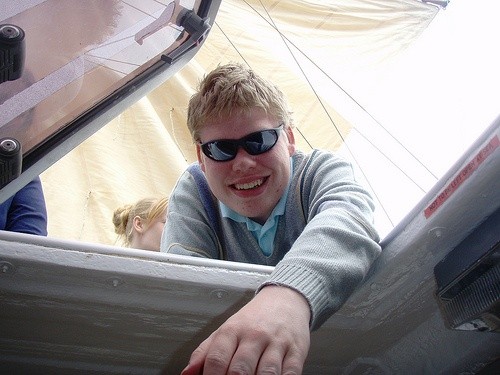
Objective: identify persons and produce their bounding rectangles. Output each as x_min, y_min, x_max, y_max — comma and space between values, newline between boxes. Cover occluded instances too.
0, 67, 49, 237
113, 194, 170, 253
160, 61, 383, 375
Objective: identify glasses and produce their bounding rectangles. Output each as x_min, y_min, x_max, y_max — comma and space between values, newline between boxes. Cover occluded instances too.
197, 123, 285, 163
